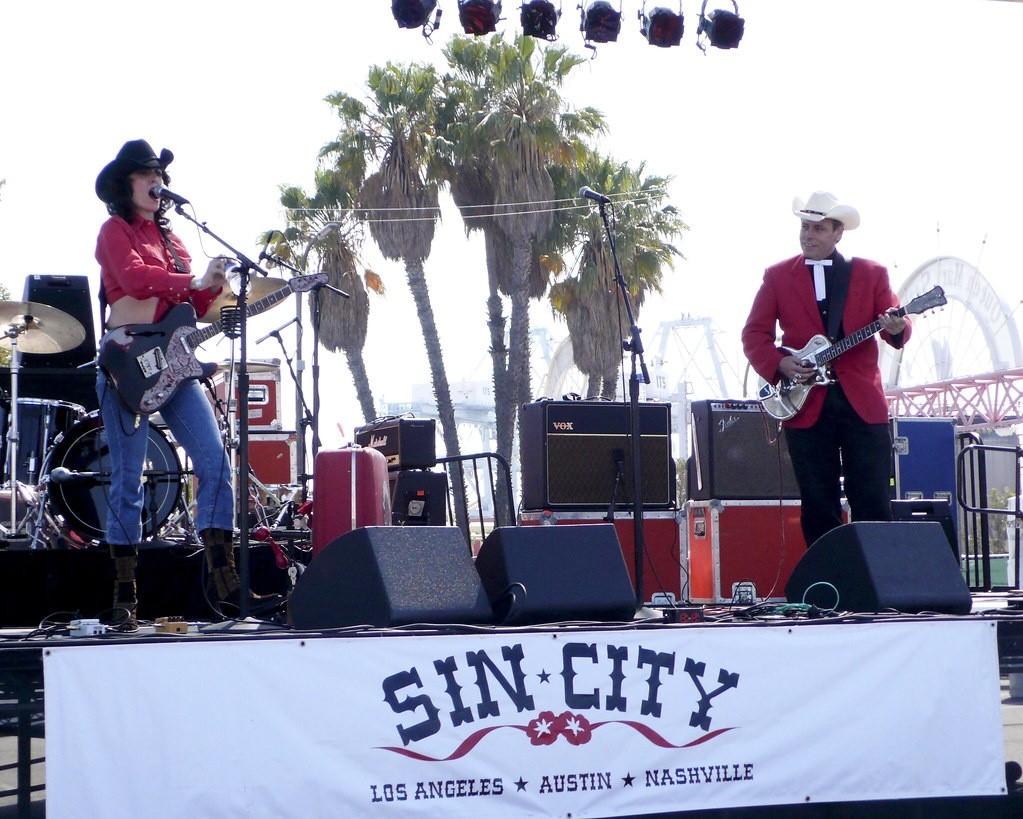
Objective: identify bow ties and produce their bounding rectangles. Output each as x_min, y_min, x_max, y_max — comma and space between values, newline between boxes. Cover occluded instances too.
804, 259, 833, 302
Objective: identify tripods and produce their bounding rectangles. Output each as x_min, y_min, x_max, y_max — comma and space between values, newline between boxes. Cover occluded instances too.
158, 375, 295, 541
0, 325, 70, 550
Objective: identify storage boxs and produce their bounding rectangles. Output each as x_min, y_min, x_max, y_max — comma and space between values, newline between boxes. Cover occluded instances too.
191, 437, 298, 521
202, 357, 284, 431
686, 497, 853, 605
518, 510, 688, 608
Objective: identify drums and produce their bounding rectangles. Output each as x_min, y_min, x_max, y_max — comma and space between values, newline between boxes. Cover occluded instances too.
42, 408, 184, 543
3, 397, 87, 503
235, 482, 310, 515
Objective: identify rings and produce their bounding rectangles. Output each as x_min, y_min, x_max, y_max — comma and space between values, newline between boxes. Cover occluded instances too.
795, 373, 801, 378
793, 378, 798, 384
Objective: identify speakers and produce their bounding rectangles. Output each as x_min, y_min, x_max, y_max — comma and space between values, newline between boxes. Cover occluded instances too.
888, 499, 960, 571
691, 399, 804, 501
387, 470, 448, 527
522, 401, 672, 509
17, 275, 97, 368
473, 523, 636, 627
286, 525, 494, 631
785, 520, 973, 616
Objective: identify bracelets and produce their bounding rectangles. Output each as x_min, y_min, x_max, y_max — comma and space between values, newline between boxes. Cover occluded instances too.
196, 279, 202, 289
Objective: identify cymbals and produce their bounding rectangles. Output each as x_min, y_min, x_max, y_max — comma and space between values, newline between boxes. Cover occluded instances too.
0, 299, 88, 356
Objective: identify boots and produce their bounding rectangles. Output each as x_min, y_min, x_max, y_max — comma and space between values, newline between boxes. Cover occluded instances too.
110, 545, 140, 632
201, 530, 283, 623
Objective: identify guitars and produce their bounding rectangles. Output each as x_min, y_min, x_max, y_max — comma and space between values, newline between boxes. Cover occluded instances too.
756, 284, 949, 422
99, 271, 332, 416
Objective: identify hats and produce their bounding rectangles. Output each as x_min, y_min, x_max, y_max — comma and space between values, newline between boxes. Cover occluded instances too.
95, 139, 174, 204
792, 191, 860, 230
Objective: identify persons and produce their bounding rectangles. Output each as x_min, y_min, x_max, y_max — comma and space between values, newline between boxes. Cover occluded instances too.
95, 139, 280, 630
742, 192, 911, 550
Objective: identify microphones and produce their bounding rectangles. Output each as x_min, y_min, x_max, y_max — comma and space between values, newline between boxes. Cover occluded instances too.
154, 184, 189, 205
51, 467, 72, 483
612, 446, 626, 481
257, 232, 273, 265
256, 317, 297, 344
579, 186, 610, 202
